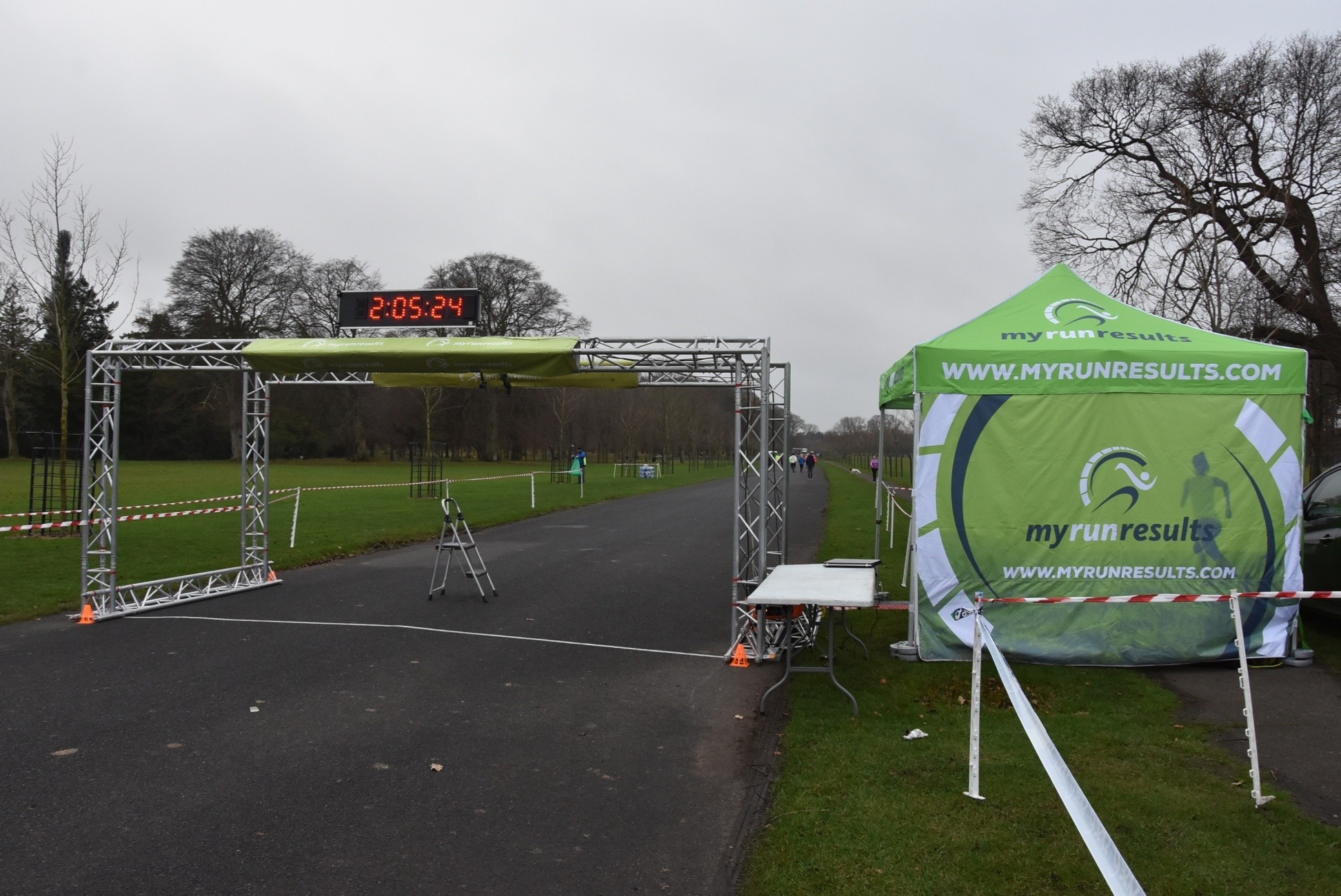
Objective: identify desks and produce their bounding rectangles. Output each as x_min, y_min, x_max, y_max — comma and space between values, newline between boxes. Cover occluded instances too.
746, 562, 875, 714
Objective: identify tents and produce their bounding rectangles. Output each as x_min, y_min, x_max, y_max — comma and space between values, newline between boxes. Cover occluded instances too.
875, 262, 1314, 670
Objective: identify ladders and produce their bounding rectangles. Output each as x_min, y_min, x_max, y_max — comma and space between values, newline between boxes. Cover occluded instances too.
429, 498, 498, 603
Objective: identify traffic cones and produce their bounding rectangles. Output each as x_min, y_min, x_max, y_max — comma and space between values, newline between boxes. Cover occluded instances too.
76, 604, 97, 625
728, 640, 752, 667
268, 570, 277, 581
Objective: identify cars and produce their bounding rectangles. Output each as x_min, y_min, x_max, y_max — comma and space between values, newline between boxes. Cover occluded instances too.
1300, 461, 1340, 620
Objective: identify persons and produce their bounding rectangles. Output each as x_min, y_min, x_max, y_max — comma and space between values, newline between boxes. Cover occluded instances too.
849, 468, 861, 475
870, 455, 879, 482
789, 451, 817, 474
571, 447, 586, 484
1179, 451, 1238, 579
806, 452, 815, 480
769, 453, 783, 468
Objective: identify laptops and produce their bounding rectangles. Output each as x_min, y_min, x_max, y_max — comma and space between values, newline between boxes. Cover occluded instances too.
823, 558, 883, 568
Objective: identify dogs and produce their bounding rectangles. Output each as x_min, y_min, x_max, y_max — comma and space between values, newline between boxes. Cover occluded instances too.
849, 468, 862, 475
